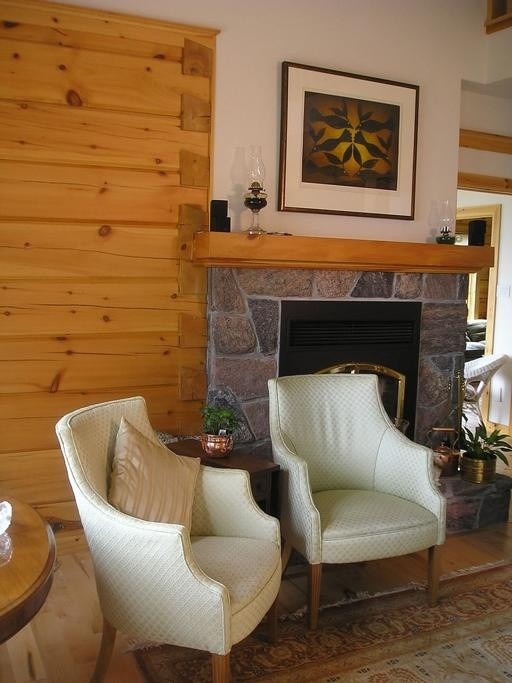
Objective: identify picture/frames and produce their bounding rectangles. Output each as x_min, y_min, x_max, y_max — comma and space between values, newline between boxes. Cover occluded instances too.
278, 61, 420, 220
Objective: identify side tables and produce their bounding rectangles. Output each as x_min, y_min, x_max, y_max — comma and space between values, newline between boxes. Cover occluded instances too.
162, 438, 281, 516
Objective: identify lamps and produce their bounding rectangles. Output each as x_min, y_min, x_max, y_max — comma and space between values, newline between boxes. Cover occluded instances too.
437, 200, 456, 245
241, 146, 269, 234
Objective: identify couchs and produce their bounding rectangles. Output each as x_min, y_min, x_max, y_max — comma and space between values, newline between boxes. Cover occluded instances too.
466, 319, 487, 342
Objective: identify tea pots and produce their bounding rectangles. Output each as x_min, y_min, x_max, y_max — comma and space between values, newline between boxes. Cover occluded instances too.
425, 428, 461, 475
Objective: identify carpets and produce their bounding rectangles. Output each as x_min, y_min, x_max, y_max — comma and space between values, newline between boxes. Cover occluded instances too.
125, 556, 512, 683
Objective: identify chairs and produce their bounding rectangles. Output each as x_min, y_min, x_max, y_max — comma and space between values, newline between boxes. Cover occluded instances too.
55, 396, 282, 683
267, 374, 448, 630
464, 353, 509, 425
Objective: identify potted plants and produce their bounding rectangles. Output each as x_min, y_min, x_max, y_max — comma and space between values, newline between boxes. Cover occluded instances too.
200, 405, 242, 459
458, 421, 512, 484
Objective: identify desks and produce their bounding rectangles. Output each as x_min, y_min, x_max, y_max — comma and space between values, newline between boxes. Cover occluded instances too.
0, 494, 57, 645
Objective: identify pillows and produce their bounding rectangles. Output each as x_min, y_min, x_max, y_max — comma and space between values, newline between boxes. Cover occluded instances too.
108, 416, 201, 532
